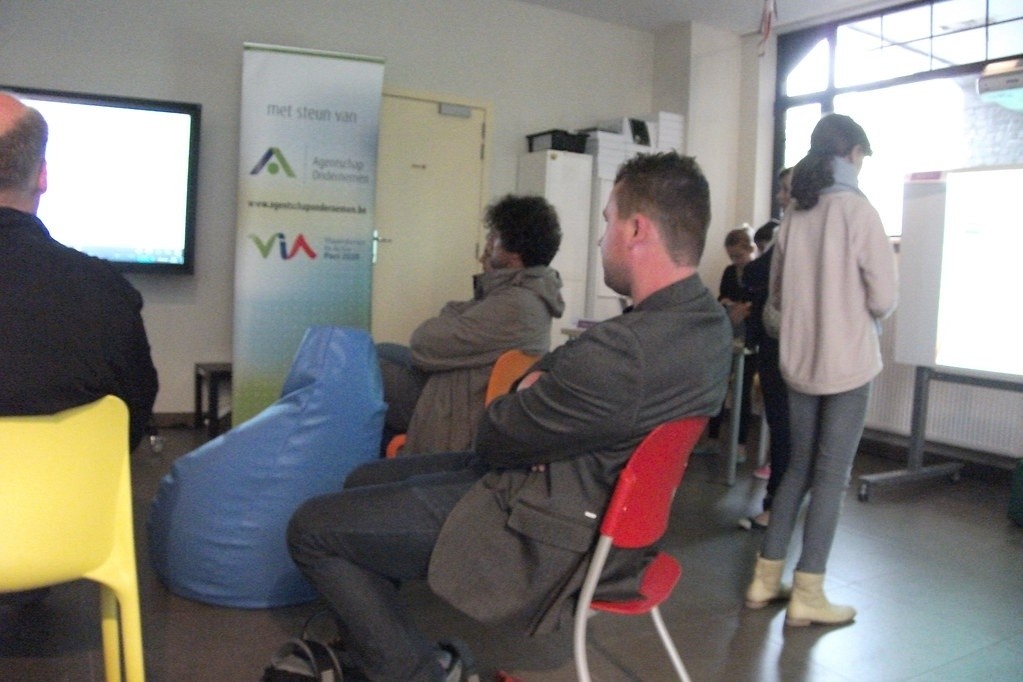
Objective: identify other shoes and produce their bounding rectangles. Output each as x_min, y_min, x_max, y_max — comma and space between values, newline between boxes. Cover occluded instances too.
739, 515, 767, 532
736, 451, 746, 464
694, 444, 720, 454
752, 464, 771, 480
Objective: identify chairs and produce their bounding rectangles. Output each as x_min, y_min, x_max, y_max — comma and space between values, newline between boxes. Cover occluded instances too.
487, 414, 713, 682
0, 395, 145, 682
386, 347, 551, 462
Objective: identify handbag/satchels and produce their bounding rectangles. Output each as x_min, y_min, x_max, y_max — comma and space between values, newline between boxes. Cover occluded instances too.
261, 611, 479, 682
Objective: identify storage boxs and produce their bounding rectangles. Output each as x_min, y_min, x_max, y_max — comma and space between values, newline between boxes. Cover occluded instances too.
516, 110, 682, 353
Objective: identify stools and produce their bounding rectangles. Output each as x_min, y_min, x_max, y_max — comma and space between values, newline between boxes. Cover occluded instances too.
193, 364, 235, 443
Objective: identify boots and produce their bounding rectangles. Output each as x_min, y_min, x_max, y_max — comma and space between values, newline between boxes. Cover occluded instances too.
786, 572, 856, 628
745, 552, 792, 608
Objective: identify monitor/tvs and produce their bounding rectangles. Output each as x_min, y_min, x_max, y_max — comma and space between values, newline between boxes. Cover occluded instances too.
0, 86, 203, 276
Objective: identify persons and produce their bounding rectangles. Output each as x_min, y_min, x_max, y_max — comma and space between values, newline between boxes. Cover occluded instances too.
287, 152, 734, 682
379, 196, 565, 456
745, 114, 900, 627
717, 165, 795, 531
0, 91, 159, 658
